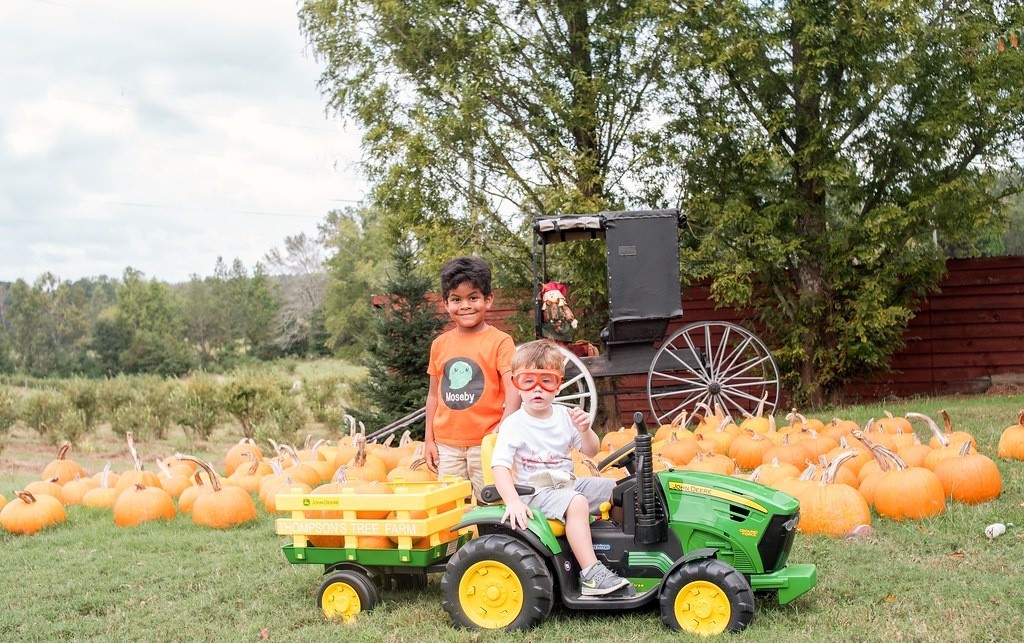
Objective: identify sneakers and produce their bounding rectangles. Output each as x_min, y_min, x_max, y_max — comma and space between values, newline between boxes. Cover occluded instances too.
580, 560, 632, 594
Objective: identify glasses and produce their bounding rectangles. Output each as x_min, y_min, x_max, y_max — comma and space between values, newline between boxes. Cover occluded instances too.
511, 370, 563, 392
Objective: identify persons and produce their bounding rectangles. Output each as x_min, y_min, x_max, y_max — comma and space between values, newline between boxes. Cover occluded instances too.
490, 339, 631, 596
423, 258, 515, 509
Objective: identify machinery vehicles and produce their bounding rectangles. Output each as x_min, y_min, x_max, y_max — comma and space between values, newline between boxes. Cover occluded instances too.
270, 409, 822, 642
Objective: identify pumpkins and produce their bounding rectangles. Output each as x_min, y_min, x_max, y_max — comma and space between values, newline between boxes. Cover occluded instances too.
0, 408, 1024, 551
